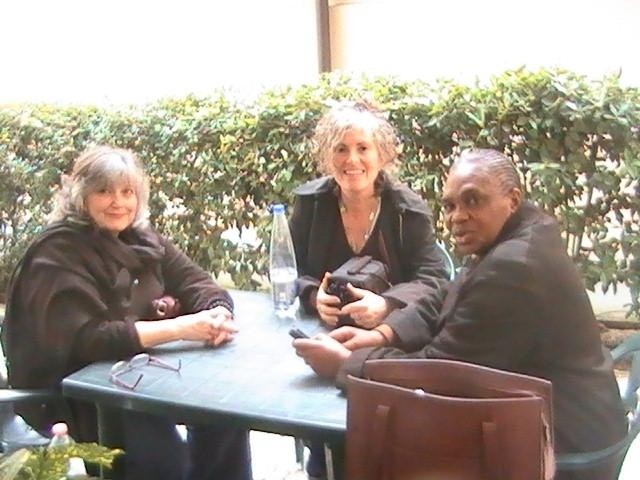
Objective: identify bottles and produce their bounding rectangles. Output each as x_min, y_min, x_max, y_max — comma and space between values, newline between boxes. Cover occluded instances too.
48, 422, 87, 479
269, 205, 301, 315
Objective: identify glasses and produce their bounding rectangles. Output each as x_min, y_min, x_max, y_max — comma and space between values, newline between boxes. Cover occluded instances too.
107, 352, 182, 390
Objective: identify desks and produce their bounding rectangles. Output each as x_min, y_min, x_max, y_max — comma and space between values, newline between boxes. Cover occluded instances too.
58, 286, 350, 479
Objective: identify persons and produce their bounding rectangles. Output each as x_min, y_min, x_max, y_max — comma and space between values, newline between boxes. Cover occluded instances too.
286, 95, 451, 478
291, 146, 630, 454
0, 145, 250, 480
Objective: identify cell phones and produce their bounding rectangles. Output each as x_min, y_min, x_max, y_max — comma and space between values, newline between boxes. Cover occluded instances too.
289, 324, 311, 337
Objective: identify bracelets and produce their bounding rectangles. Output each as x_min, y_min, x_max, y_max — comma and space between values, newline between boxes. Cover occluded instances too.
374, 327, 389, 345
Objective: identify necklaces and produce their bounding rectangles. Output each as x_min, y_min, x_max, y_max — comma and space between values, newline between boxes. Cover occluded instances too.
339, 195, 378, 252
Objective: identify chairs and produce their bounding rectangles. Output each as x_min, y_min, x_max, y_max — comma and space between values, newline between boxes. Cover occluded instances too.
553, 332, 640, 480
0, 313, 124, 478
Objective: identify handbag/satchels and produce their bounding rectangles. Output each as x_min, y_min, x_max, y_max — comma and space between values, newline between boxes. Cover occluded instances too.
343, 358, 556, 480
326, 256, 389, 326
